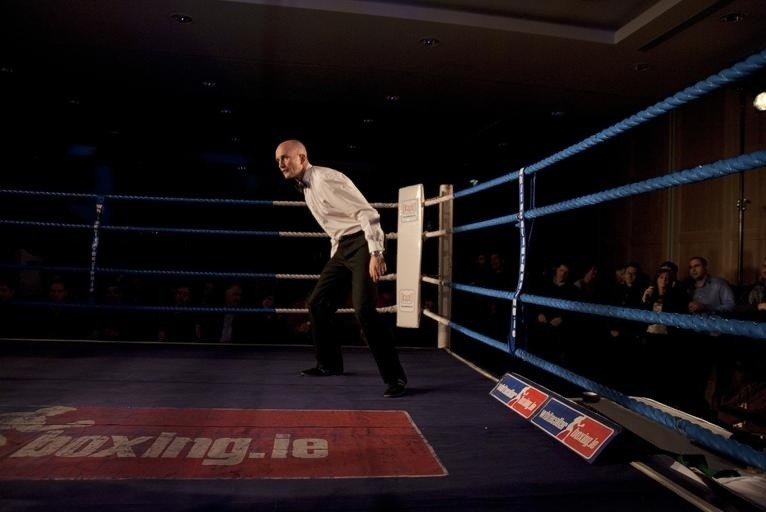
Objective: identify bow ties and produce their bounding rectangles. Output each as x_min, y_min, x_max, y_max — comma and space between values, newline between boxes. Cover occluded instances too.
295, 182, 310, 192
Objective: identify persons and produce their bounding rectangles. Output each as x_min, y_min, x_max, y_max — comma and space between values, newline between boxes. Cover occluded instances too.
274, 139, 409, 398
0, 251, 399, 351
422, 252, 765, 450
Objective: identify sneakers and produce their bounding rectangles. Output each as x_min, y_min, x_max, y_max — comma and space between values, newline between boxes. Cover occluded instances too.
302, 365, 343, 376
384, 377, 407, 396
